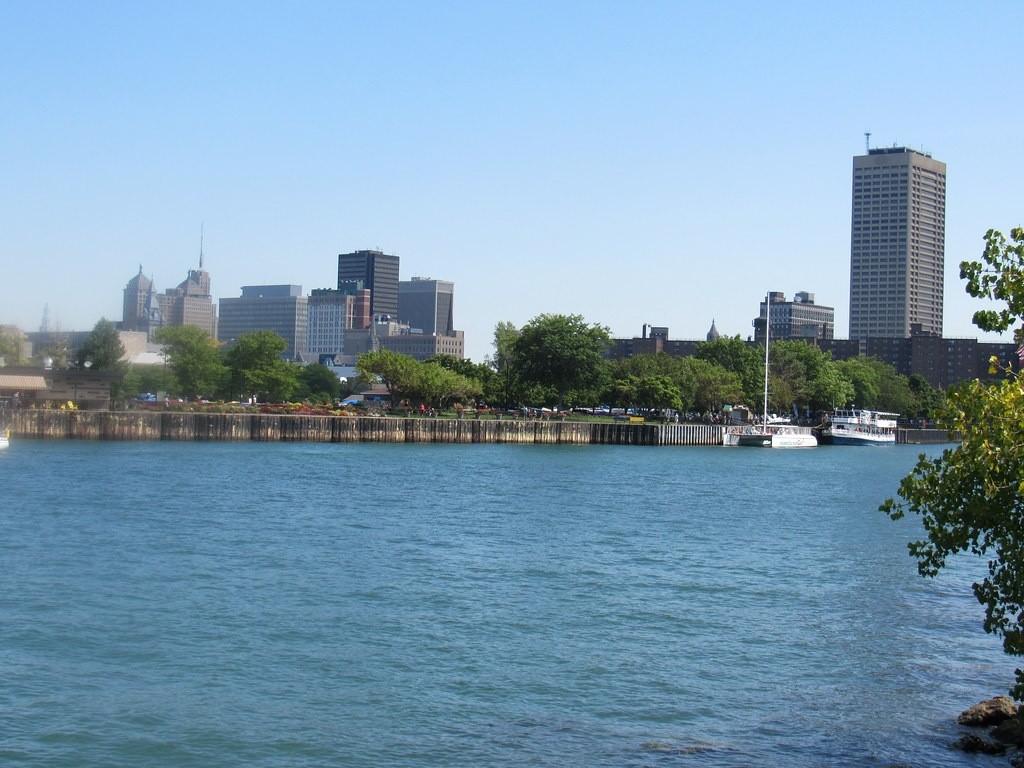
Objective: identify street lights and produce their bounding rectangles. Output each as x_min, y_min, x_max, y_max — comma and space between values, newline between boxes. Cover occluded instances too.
228, 336, 244, 404
497, 352, 508, 410
73, 360, 96, 408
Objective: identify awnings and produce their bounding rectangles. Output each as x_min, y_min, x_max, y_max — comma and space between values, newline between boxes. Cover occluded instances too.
0, 375, 48, 391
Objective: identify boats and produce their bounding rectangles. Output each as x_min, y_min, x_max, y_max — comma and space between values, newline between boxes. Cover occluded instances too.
0, 427, 9, 451
820, 404, 900, 446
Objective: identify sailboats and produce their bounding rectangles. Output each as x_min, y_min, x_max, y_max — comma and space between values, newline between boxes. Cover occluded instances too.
740, 291, 819, 448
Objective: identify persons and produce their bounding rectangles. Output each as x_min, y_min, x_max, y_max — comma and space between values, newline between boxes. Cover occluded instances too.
13, 393, 26, 411
418, 402, 432, 416
674, 412, 731, 425
253, 395, 257, 407
163, 394, 170, 411
